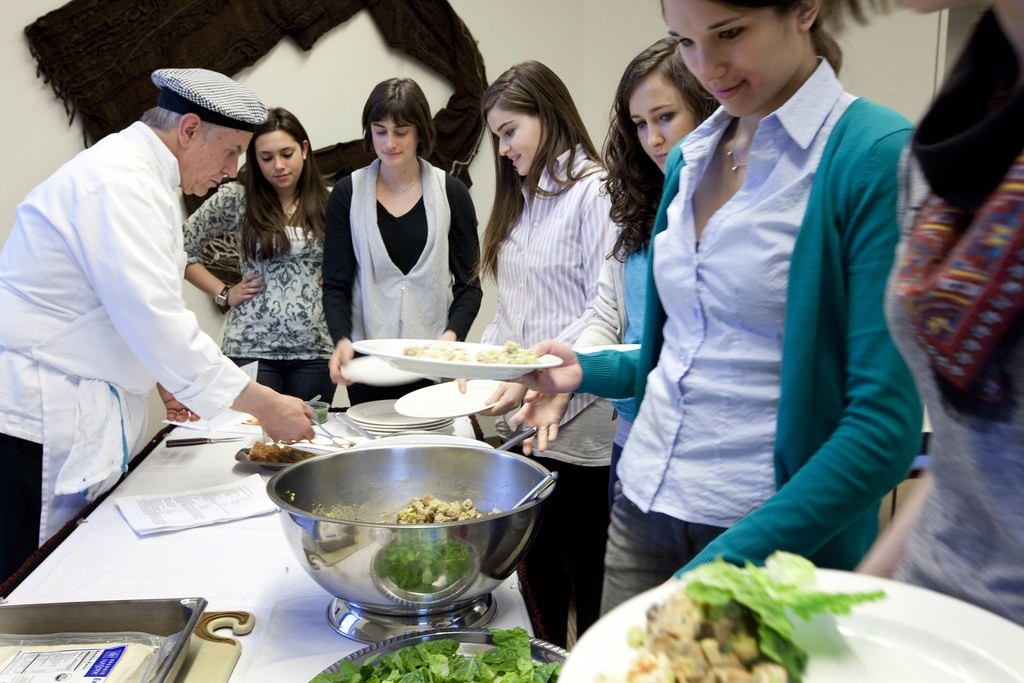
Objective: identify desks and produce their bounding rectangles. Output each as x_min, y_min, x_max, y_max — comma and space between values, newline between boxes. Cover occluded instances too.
0, 406, 540, 683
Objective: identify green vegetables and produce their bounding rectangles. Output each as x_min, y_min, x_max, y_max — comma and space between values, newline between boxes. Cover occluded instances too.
310, 628, 562, 683
376, 541, 472, 593
688, 554, 886, 682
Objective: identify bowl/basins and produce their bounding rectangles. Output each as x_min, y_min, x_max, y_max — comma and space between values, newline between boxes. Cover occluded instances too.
297, 401, 331, 424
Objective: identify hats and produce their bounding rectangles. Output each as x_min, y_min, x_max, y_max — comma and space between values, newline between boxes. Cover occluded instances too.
151, 68, 270, 134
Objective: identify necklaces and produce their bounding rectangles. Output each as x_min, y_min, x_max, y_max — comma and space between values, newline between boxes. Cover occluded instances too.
727, 132, 748, 174
378, 174, 420, 194
280, 200, 294, 216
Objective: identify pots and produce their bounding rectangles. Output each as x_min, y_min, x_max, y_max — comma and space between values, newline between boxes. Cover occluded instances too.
267, 443, 560, 645
305, 627, 574, 683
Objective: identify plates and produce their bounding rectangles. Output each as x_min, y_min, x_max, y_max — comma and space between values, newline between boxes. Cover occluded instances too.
351, 435, 495, 452
556, 562, 1024, 683
395, 380, 510, 418
346, 399, 456, 441
235, 443, 348, 466
350, 339, 566, 380
341, 357, 423, 386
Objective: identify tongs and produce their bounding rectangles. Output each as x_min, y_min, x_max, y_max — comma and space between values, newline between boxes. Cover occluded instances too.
304, 420, 356, 451
495, 422, 538, 451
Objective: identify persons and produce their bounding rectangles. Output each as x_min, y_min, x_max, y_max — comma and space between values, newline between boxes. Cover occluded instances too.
1, 69, 316, 603
840, 0, 1023, 627
458, 0, 916, 623
182, 108, 329, 402
322, 77, 483, 404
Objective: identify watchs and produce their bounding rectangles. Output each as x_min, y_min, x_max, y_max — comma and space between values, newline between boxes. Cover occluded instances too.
215, 285, 230, 315
